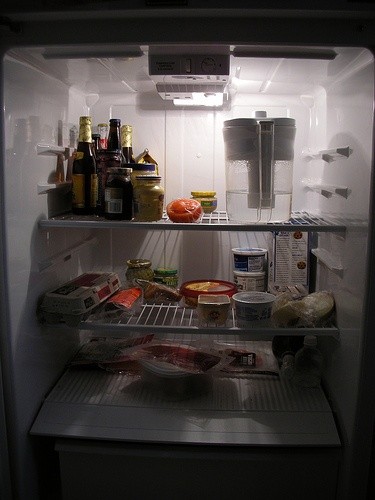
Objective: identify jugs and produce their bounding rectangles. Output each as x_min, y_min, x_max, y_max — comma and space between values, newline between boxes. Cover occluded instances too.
222, 109, 297, 224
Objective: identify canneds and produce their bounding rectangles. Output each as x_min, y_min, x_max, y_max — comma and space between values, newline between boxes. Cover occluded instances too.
103, 168, 135, 221
152, 268, 178, 288
190, 191, 217, 213
131, 163, 163, 222
125, 258, 152, 289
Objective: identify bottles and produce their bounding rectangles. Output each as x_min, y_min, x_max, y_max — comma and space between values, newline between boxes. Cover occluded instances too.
70, 115, 99, 215
91, 123, 134, 163
99, 118, 126, 219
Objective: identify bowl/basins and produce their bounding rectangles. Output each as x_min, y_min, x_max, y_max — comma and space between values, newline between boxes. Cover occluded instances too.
136, 357, 205, 395
181, 280, 237, 309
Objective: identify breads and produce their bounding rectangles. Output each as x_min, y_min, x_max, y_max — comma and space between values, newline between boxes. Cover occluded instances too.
270, 290, 335, 328
143, 282, 182, 303
